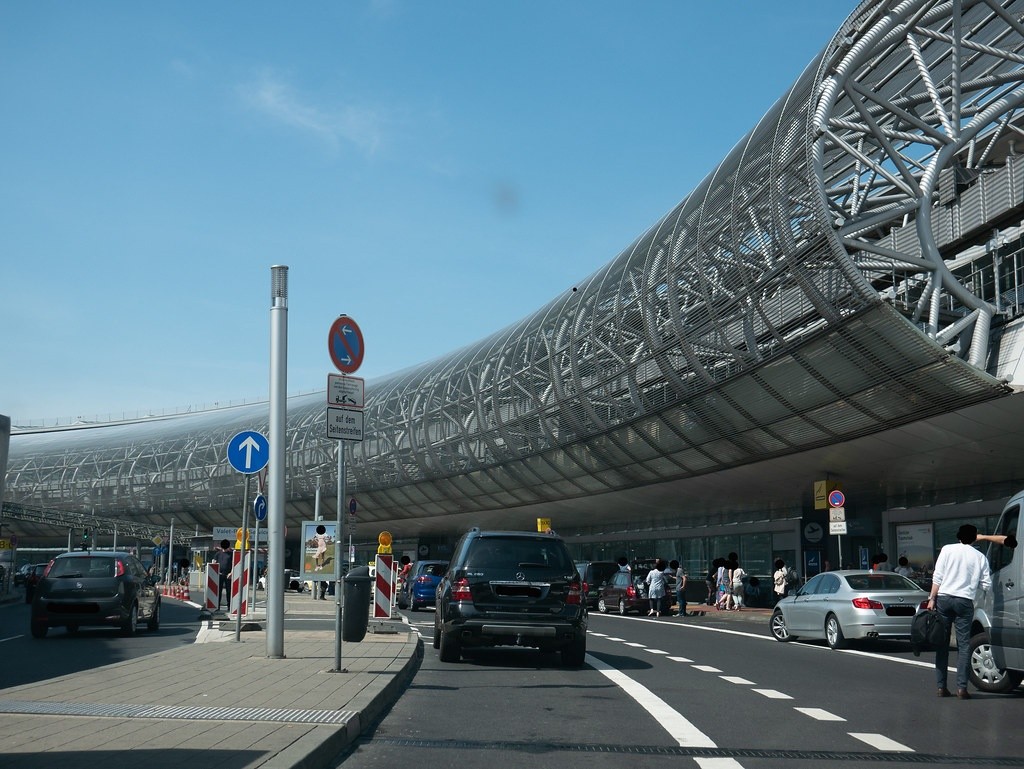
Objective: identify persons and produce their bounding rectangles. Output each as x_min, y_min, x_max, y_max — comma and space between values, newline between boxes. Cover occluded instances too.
875, 552, 896, 572
398, 555, 413, 580
705, 552, 746, 613
927, 525, 993, 701
975, 533, 1018, 548
645, 558, 668, 617
311, 525, 329, 571
212, 539, 233, 612
893, 557, 918, 580
668, 560, 688, 617
774, 560, 789, 603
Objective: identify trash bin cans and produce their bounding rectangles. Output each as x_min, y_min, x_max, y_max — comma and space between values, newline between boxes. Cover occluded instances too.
343, 565, 369, 642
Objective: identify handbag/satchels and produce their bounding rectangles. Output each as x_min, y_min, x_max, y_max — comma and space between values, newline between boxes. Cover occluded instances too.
774, 576, 783, 585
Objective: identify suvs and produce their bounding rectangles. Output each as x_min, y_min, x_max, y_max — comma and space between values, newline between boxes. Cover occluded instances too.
432, 526, 588, 669
573, 559, 621, 611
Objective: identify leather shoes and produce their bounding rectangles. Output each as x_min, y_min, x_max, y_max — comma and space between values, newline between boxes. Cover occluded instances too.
958, 689, 971, 700
938, 688, 951, 697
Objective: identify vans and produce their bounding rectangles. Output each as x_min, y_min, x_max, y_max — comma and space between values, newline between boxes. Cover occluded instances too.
966, 490, 1024, 694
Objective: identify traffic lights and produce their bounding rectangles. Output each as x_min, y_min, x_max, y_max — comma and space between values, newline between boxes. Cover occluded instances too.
82, 527, 89, 540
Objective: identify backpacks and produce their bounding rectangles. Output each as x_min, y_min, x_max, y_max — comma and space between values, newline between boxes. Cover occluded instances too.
910, 608, 946, 657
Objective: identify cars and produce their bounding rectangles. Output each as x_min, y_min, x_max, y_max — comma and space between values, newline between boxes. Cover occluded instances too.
12, 562, 49, 605
398, 559, 452, 612
770, 568, 934, 650
597, 568, 672, 615
283, 562, 401, 603
32, 550, 161, 638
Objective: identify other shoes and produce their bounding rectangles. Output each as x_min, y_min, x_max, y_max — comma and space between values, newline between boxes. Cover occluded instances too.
657, 614, 661, 617
673, 614, 684, 618
648, 612, 654, 617
320, 596, 328, 600
